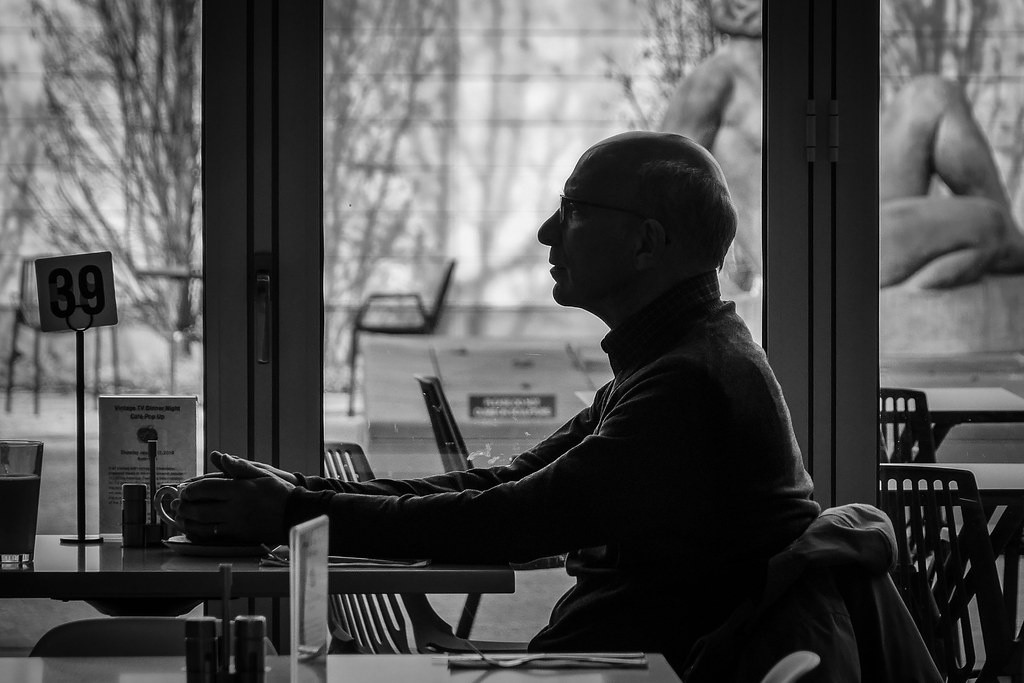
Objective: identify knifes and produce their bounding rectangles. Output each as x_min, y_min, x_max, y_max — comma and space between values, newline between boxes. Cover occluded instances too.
431, 652, 647, 667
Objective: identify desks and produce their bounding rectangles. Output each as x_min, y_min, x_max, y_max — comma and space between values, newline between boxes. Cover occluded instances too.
879, 461, 1024, 620
879, 385, 1024, 463
0, 652, 680, 683
0, 534, 516, 655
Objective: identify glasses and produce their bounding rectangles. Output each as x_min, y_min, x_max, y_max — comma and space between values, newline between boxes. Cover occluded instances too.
558, 195, 641, 228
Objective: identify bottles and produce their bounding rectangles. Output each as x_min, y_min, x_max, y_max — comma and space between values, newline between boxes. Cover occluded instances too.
121, 483, 148, 549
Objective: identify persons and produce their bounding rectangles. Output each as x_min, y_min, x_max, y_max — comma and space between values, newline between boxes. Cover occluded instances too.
654, 0, 1023, 292
171, 130, 818, 683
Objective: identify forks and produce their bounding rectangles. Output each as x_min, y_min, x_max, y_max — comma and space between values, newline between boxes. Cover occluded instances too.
464, 640, 649, 668
261, 543, 432, 564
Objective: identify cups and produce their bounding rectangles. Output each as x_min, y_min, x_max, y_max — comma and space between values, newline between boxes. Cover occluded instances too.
153, 482, 191, 537
0, 441, 44, 566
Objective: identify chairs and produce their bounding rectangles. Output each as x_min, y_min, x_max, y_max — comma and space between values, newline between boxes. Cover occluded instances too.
876, 464, 1013, 683
879, 388, 948, 563
321, 441, 479, 655
412, 376, 476, 471
683, 501, 945, 683
28, 617, 279, 656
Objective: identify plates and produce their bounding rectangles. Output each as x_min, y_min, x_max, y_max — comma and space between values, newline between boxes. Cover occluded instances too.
160, 535, 262, 558
